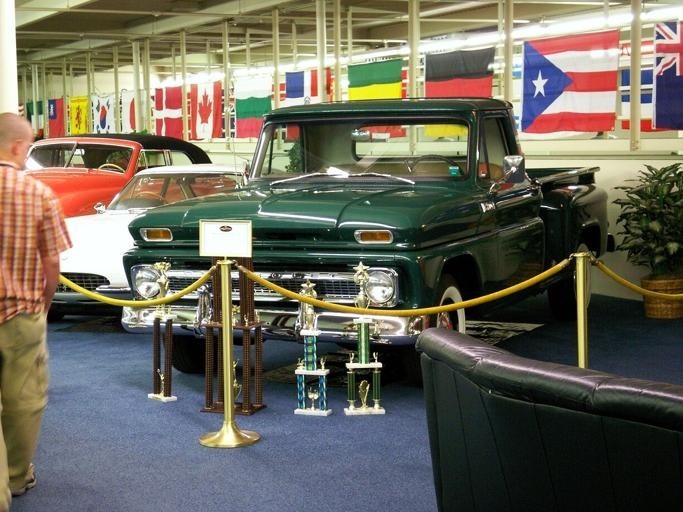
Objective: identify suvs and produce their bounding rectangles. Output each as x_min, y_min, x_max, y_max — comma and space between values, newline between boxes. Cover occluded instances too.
113, 94, 619, 380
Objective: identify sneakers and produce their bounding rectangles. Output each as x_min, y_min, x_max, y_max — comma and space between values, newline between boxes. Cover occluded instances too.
10, 472, 36, 497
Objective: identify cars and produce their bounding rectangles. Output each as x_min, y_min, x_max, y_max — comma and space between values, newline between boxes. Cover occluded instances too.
23, 131, 237, 220
45, 162, 284, 323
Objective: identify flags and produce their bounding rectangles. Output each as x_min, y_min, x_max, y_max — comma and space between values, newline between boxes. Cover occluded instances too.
347, 59, 402, 101
422, 47, 493, 137
358, 57, 409, 137
284, 67, 331, 140
232, 66, 272, 138
621, 70, 678, 134
520, 30, 616, 131
18, 79, 284, 143
653, 20, 682, 132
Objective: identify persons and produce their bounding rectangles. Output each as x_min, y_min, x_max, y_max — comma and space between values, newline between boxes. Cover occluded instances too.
0, 113, 73, 511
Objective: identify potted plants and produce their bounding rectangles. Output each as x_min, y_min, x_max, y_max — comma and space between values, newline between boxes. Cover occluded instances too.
611, 163, 683, 319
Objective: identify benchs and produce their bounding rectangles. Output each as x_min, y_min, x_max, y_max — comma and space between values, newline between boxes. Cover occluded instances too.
316, 163, 504, 182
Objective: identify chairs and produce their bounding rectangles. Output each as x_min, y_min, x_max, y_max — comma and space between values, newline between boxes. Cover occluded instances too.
414, 328, 683, 512
107, 159, 128, 172
